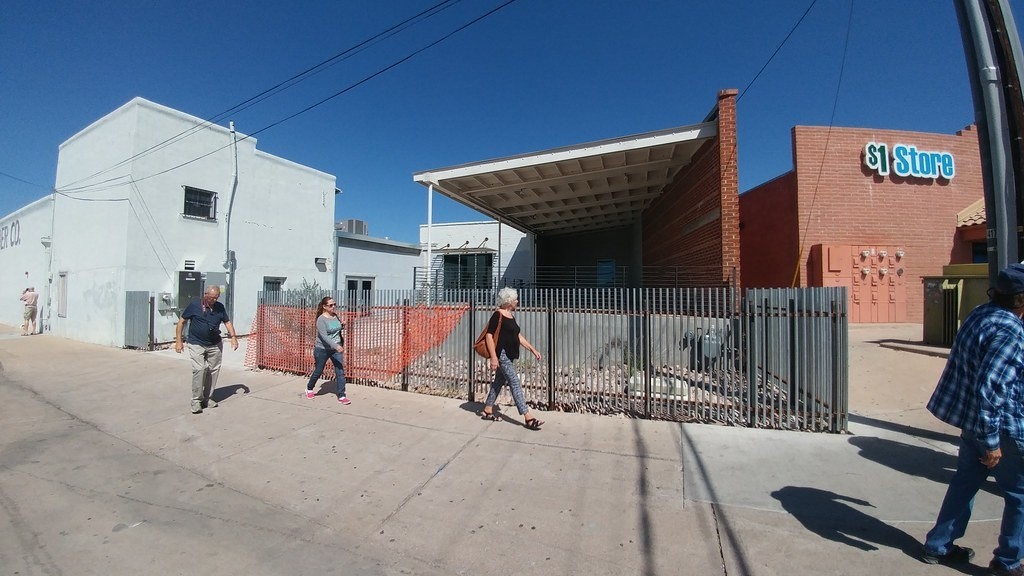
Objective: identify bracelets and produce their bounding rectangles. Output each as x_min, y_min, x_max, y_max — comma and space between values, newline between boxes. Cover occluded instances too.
231, 335, 235, 337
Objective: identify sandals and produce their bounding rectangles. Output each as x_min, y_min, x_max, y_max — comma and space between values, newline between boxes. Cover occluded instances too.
526, 418, 545, 429
482, 409, 502, 422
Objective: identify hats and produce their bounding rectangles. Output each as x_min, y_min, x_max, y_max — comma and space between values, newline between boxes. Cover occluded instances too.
994, 263, 1024, 295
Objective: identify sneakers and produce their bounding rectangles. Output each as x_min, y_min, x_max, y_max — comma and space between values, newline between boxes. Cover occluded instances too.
337, 397, 351, 405
203, 398, 218, 408
305, 386, 315, 400
191, 399, 202, 413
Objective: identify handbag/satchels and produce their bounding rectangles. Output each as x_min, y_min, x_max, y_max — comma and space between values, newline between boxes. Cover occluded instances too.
473, 311, 502, 358
334, 313, 344, 347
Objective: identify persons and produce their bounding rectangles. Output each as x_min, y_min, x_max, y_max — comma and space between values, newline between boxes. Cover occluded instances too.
482, 287, 545, 429
176, 285, 238, 414
305, 297, 351, 405
20, 286, 39, 336
920, 263, 1024, 576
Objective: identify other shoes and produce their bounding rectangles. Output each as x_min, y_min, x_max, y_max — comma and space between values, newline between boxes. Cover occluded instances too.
30, 332, 37, 335
922, 544, 975, 563
22, 332, 27, 336
987, 559, 1023, 576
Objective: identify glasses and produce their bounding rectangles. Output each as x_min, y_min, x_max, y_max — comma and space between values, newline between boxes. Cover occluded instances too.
325, 303, 336, 307
